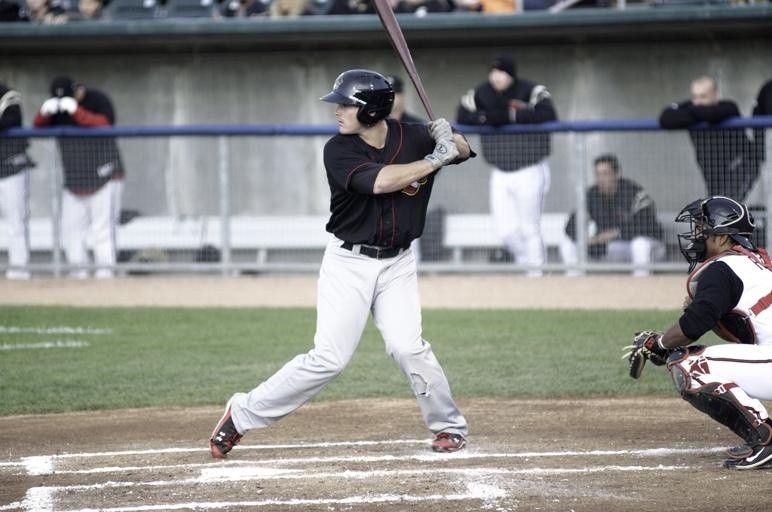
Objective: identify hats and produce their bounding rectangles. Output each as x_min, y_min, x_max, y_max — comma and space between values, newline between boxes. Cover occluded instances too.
487, 53, 517, 79
49, 75, 76, 98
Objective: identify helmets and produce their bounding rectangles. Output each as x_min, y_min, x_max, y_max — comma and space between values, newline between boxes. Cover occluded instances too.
675, 195, 755, 275
320, 69, 396, 128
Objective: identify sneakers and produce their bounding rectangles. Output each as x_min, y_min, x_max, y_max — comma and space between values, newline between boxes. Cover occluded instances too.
431, 426, 468, 453
209, 393, 253, 460
735, 419, 771, 470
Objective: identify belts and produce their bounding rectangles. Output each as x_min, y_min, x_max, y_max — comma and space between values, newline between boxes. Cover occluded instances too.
341, 242, 413, 260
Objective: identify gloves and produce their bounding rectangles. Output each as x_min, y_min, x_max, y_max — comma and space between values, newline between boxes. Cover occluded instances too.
40, 97, 59, 118
59, 96, 80, 116
425, 139, 461, 168
427, 117, 453, 144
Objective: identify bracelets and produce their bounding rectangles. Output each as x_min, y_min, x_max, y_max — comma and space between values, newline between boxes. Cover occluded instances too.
656, 334, 669, 351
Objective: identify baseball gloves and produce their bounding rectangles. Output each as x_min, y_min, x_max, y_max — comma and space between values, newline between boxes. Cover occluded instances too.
629, 332, 670, 379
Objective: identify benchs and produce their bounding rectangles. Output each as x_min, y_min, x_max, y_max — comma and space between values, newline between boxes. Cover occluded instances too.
0, 207, 772, 265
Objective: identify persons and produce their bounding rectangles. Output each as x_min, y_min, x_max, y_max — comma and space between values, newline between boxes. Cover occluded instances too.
33, 74, 125, 278
750, 81, 771, 253
658, 73, 759, 203
209, 69, 478, 459
562, 153, 668, 277
0, 84, 38, 279
455, 45, 557, 276
620, 193, 771, 470
383, 75, 444, 262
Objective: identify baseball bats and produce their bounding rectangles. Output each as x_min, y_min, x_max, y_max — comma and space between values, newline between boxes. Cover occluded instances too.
375, 0, 437, 121
